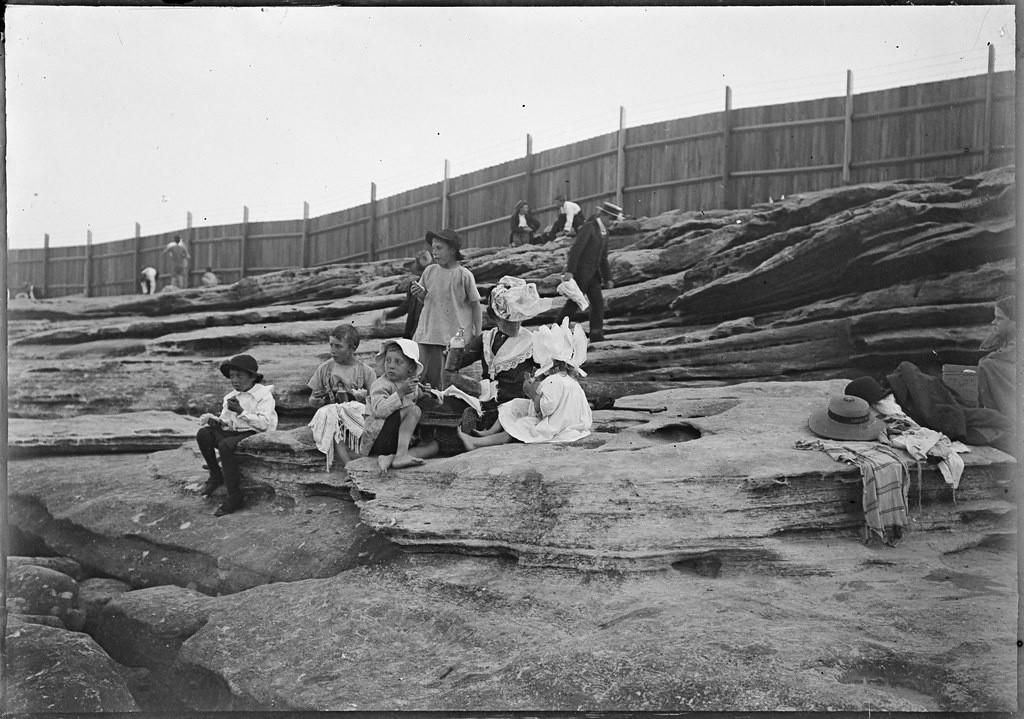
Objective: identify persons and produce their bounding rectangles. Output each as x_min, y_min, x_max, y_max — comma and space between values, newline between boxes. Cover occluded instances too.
141, 235, 219, 296
549, 195, 584, 235
307, 228, 592, 472
508, 200, 541, 248
977, 295, 1017, 421
553, 201, 626, 343
195, 355, 278, 517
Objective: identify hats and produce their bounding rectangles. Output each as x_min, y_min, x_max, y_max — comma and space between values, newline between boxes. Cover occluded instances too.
426, 228, 464, 261
844, 376, 892, 405
809, 395, 888, 441
409, 251, 437, 276
220, 355, 263, 383
533, 314, 587, 378
515, 200, 528, 213
488, 275, 552, 323
596, 201, 625, 219
375, 338, 423, 379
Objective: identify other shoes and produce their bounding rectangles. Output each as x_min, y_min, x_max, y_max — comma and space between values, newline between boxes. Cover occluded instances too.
201, 473, 223, 495
214, 496, 245, 515
